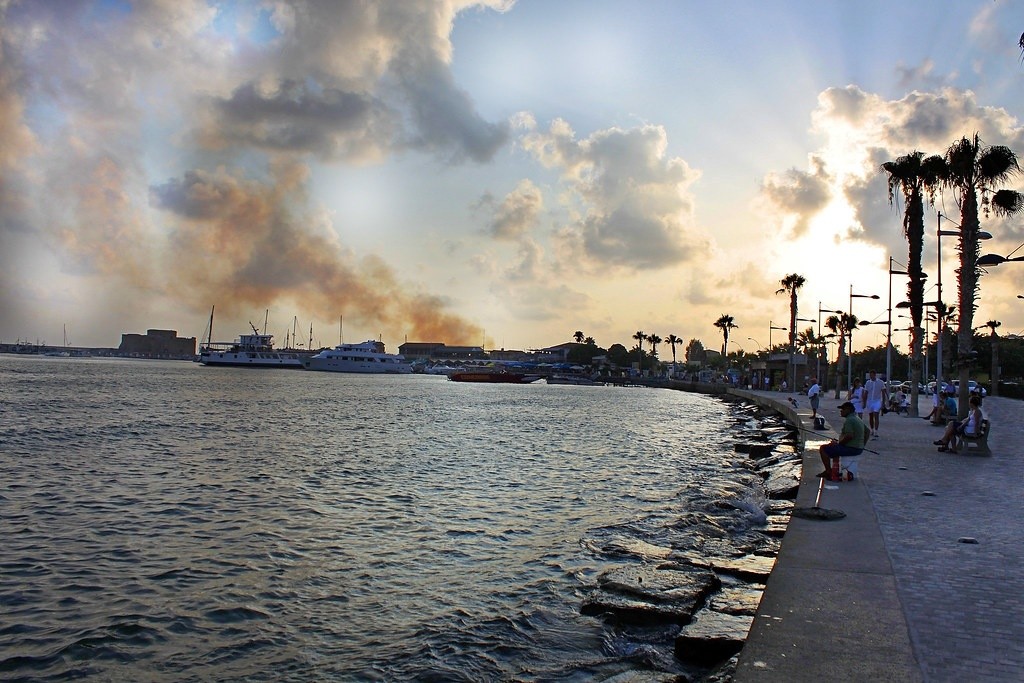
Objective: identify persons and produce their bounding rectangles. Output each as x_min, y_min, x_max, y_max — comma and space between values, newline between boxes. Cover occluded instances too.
943, 380, 956, 398
733, 374, 736, 384
922, 389, 957, 425
891, 386, 909, 416
782, 380, 787, 388
764, 375, 770, 391
739, 373, 743, 384
929, 378, 937, 406
815, 402, 871, 478
807, 378, 820, 418
973, 382, 982, 406
743, 376, 749, 389
752, 375, 757, 391
788, 397, 799, 408
864, 369, 887, 436
933, 396, 983, 454
847, 376, 868, 420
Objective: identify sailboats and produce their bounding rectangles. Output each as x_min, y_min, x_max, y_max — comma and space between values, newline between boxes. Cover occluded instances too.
193, 305, 306, 363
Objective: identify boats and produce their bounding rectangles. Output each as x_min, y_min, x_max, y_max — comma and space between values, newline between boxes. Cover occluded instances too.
199, 322, 301, 367
306, 341, 414, 374
450, 369, 540, 383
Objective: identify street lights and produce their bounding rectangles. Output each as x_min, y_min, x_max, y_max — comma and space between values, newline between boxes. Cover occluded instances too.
858, 320, 890, 381
731, 341, 742, 352
886, 271, 928, 407
748, 338, 760, 352
770, 327, 787, 355
848, 295, 880, 391
935, 230, 994, 422
895, 301, 942, 382
817, 309, 843, 384
794, 318, 817, 391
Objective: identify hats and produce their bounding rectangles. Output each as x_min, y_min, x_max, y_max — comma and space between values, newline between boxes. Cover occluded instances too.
837, 402, 855, 410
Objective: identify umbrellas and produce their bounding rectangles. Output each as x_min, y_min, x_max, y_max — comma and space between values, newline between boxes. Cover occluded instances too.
522, 362, 584, 369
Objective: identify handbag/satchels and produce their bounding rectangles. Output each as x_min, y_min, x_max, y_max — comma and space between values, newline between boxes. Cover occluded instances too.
814, 418, 825, 430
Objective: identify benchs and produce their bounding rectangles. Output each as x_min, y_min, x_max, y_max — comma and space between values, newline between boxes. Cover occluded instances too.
957, 418, 991, 455
941, 408, 961, 426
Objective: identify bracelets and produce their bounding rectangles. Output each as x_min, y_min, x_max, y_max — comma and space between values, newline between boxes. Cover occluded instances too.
837, 441, 839, 444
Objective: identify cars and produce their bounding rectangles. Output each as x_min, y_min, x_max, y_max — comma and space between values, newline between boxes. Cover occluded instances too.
900, 381, 924, 394
952, 379, 987, 396
924, 382, 950, 394
885, 381, 902, 392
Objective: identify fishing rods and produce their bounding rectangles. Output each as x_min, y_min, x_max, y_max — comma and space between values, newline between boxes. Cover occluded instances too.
767, 417, 880, 456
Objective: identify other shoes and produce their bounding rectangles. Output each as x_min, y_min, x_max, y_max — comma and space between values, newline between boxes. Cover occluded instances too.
816, 471, 833, 481
938, 446, 949, 452
874, 431, 879, 437
923, 417, 930, 420
810, 416, 816, 419
870, 429, 874, 434
931, 421, 935, 424
946, 448, 958, 454
934, 440, 947, 445
